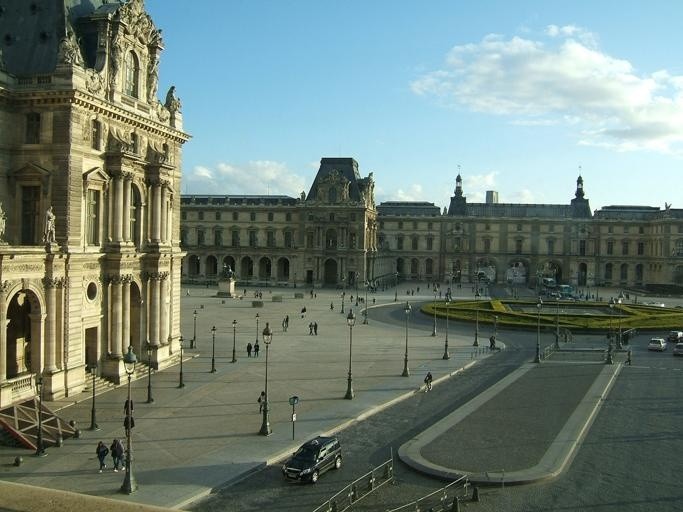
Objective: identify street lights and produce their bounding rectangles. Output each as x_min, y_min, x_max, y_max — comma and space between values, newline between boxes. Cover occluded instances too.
31, 377, 48, 457
442, 270, 624, 364
337, 264, 398, 325
260, 322, 276, 437
118, 345, 138, 496
343, 309, 357, 400
87, 360, 100, 431
430, 282, 438, 337
400, 300, 413, 377
146, 309, 259, 404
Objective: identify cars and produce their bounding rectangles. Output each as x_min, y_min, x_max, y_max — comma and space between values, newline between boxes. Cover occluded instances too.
673, 304, 682, 308
648, 301, 664, 308
672, 343, 682, 356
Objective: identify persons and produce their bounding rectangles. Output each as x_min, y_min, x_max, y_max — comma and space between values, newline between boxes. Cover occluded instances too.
244, 282, 450, 357
42, 206, 56, 243
96, 440, 126, 473
490, 336, 495, 348
258, 391, 266, 413
625, 350, 631, 365
124, 416, 135, 436
425, 372, 432, 392
124, 396, 133, 415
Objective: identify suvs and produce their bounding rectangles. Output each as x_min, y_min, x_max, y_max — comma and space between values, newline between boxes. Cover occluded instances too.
667, 330, 682, 342
647, 336, 667, 352
279, 436, 343, 484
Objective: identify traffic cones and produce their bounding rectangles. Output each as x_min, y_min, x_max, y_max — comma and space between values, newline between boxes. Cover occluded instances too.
381, 464, 389, 479
471, 485, 479, 502
352, 485, 358, 501
451, 496, 460, 512
367, 476, 371, 491
429, 508, 435, 512
332, 499, 339, 512
